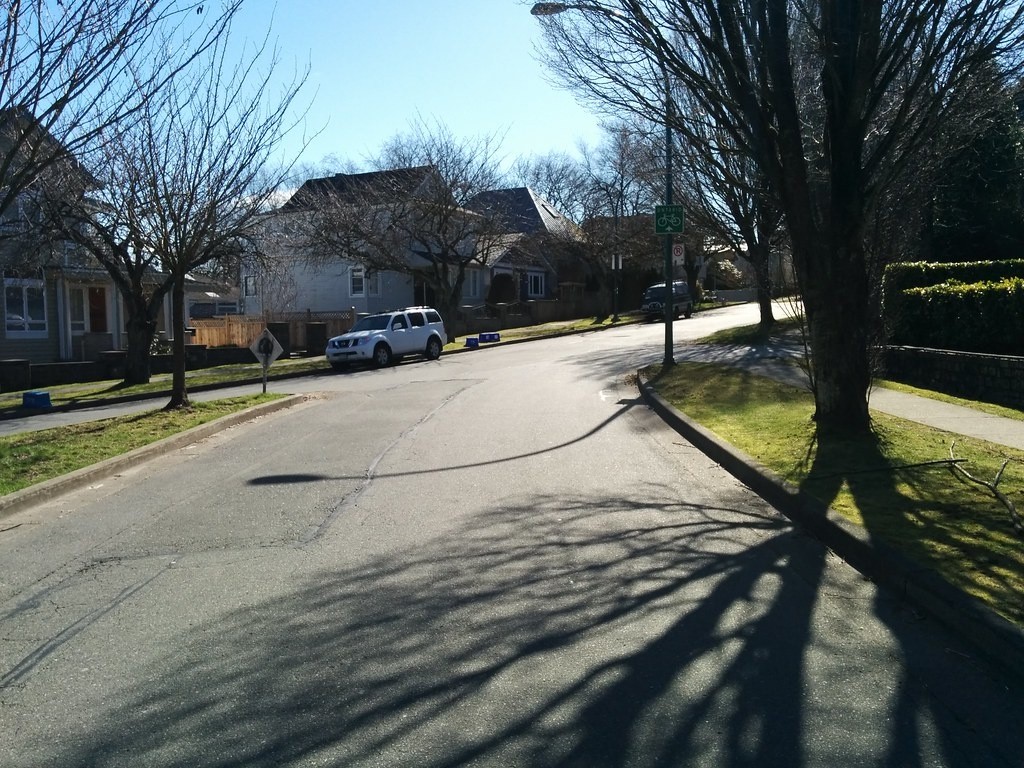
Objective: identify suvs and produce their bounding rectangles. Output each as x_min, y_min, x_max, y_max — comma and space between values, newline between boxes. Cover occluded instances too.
326, 306, 449, 372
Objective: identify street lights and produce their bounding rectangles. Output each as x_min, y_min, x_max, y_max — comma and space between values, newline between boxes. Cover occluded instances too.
529, 2, 676, 367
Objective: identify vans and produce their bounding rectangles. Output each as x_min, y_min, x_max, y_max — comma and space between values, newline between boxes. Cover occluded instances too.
641, 280, 694, 322
